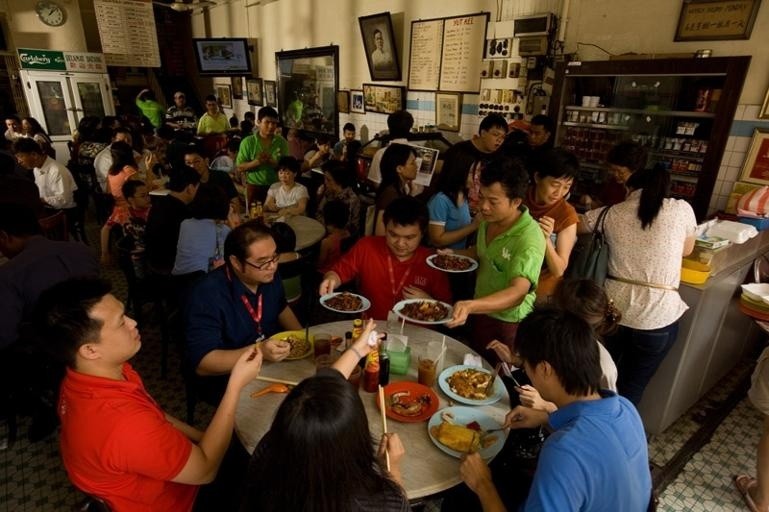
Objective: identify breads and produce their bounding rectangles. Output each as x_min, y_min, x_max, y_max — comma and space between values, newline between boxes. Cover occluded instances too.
429, 422, 500, 454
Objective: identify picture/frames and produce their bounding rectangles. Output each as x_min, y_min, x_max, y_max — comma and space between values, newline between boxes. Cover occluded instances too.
245, 77, 263, 106
433, 90, 463, 132
338, 90, 350, 114
349, 88, 366, 114
231, 77, 244, 99
214, 83, 233, 109
362, 83, 407, 116
264, 79, 277, 107
357, 10, 403, 82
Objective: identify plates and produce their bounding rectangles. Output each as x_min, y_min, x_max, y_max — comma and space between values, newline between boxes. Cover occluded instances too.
319, 292, 371, 313
737, 294, 769, 322
268, 330, 315, 361
439, 364, 506, 406
375, 381, 440, 422
393, 298, 457, 324
428, 406, 504, 460
426, 254, 479, 274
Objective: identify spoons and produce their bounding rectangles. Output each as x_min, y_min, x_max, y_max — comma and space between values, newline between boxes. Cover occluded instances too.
304, 323, 310, 352
250, 383, 289, 399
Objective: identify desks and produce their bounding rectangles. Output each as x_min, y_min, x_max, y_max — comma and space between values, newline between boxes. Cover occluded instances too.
223, 212, 328, 258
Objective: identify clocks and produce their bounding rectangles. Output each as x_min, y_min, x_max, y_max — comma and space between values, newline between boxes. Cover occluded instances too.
35, 0, 65, 26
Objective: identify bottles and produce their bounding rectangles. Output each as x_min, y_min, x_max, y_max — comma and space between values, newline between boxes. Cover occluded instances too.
250, 201, 263, 218
341, 330, 355, 357
378, 331, 392, 385
352, 318, 363, 339
418, 125, 434, 133
366, 345, 380, 392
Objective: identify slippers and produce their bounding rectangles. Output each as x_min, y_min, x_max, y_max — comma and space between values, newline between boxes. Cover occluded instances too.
734, 474, 765, 511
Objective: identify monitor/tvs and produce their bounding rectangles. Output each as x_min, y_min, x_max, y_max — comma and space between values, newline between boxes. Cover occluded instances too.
192, 38, 253, 78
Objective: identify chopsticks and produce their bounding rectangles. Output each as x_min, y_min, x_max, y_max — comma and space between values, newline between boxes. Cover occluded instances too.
254, 374, 299, 387
376, 382, 394, 474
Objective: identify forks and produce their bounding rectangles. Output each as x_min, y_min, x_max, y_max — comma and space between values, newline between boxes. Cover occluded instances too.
502, 361, 523, 389
479, 424, 510, 438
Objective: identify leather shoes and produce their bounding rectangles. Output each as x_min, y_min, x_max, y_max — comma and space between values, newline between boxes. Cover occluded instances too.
26, 407, 61, 445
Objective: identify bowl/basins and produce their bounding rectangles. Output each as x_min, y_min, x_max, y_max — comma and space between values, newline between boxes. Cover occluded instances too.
681, 259, 712, 285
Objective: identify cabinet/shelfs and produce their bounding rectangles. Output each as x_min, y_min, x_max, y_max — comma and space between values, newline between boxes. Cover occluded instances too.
17, 68, 116, 170
551, 55, 754, 223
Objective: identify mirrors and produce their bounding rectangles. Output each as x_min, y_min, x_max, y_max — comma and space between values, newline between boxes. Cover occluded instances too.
275, 44, 339, 143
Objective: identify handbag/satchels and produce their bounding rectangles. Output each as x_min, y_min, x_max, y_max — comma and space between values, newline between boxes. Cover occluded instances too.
565, 204, 611, 289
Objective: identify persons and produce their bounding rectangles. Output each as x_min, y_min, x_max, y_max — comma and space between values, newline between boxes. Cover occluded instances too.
732, 346, 768, 512
370, 27, 395, 71
1, 86, 701, 511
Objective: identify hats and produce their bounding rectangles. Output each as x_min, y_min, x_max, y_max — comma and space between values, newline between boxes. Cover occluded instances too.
174, 92, 184, 99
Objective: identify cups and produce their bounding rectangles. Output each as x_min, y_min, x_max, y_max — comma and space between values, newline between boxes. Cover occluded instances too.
418, 351, 439, 385
348, 365, 361, 395
312, 331, 333, 353
426, 339, 447, 369
314, 354, 334, 376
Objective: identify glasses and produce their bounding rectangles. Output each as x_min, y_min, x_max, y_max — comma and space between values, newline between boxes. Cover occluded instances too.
605, 167, 631, 179
133, 194, 150, 199
183, 160, 201, 167
243, 251, 280, 272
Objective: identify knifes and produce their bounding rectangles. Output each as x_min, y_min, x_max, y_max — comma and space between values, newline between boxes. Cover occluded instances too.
485, 361, 503, 394
